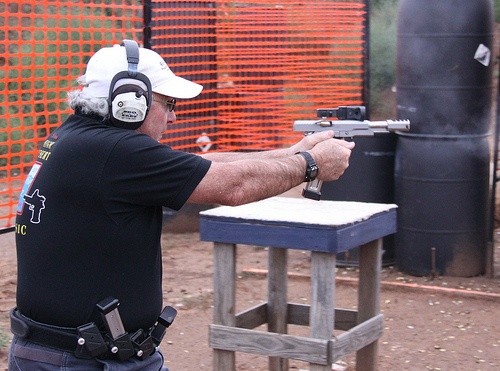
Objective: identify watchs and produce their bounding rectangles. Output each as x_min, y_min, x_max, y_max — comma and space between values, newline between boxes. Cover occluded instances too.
294, 151, 320, 183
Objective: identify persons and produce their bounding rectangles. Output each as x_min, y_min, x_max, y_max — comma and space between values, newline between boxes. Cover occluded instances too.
5, 39, 357, 371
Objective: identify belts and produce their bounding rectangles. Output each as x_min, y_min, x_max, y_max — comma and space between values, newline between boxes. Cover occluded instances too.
9, 307, 164, 361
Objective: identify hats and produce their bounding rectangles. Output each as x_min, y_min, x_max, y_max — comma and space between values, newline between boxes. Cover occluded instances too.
81, 44, 204, 99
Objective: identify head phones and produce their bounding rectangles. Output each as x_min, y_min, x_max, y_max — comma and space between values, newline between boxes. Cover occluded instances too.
104, 39, 154, 130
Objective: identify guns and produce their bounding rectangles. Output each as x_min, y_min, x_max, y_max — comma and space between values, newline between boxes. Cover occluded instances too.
292, 104, 411, 202
